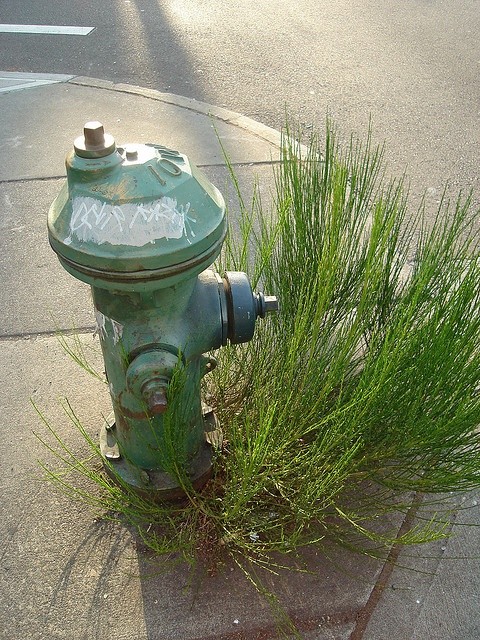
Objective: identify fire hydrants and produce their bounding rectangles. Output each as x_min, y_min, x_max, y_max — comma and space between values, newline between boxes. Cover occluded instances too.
47, 121, 278, 503
